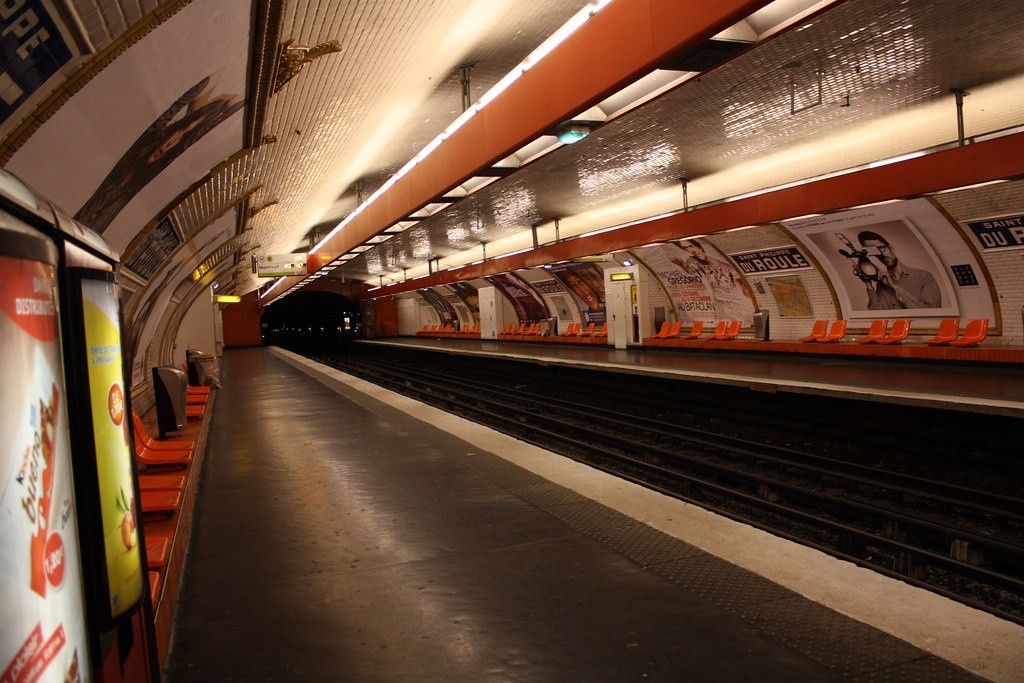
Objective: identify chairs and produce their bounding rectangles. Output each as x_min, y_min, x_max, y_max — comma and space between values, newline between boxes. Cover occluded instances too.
461, 323, 471, 333
597, 322, 609, 338
472, 324, 479, 333
505, 323, 518, 334
580, 322, 596, 337
437, 323, 444, 333
855, 318, 889, 344
141, 535, 170, 610
423, 323, 428, 332
704, 321, 729, 340
137, 475, 188, 518
924, 317, 961, 347
430, 323, 437, 332
680, 320, 703, 340
445, 324, 451, 333
650, 321, 672, 339
659, 321, 682, 339
562, 322, 575, 336
523, 323, 535, 335
816, 319, 848, 343
569, 322, 582, 336
716, 320, 742, 341
876, 319, 913, 346
184, 385, 212, 419
129, 407, 197, 466
799, 318, 828, 343
529, 324, 540, 336
950, 318, 989, 347
511, 323, 526, 335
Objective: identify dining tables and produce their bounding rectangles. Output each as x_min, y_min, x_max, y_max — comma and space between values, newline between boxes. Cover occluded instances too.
500, 323, 511, 334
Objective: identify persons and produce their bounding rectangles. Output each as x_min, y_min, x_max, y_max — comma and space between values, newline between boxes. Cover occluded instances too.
848, 230, 943, 308
667, 240, 740, 322
552, 263, 604, 312
68, 66, 242, 237
